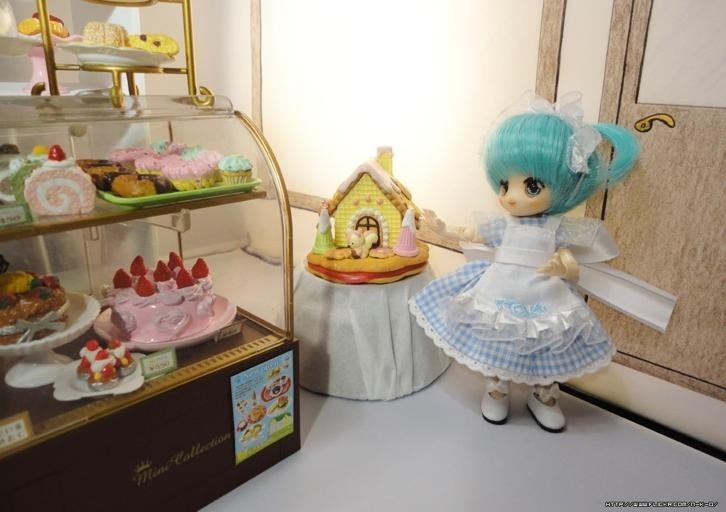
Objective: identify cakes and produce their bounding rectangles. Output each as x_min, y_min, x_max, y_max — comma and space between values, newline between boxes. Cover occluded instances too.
304, 147, 429, 284
93, 252, 236, 352
0, 270, 68, 345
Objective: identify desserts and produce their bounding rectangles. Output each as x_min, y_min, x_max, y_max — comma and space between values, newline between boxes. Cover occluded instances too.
0, 138, 97, 223
74, 337, 137, 391
76, 139, 255, 198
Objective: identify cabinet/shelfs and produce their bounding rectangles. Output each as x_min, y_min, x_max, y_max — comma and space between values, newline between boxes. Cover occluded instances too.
0, 111, 301, 512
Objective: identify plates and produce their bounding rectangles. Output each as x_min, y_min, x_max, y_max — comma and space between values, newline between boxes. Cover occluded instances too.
52, 354, 152, 404
57, 42, 173, 64
